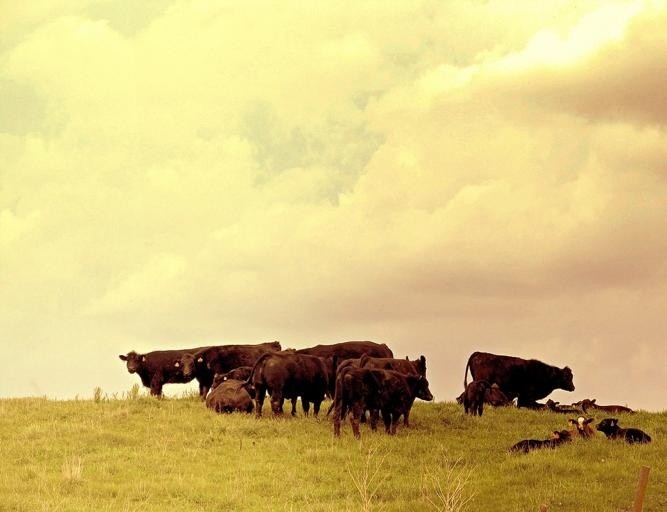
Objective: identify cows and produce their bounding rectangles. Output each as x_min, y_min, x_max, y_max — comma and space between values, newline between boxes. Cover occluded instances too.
597, 416, 652, 446
117, 338, 434, 440
567, 415, 595, 441
461, 350, 575, 409
456, 378, 635, 417
510, 428, 575, 453
